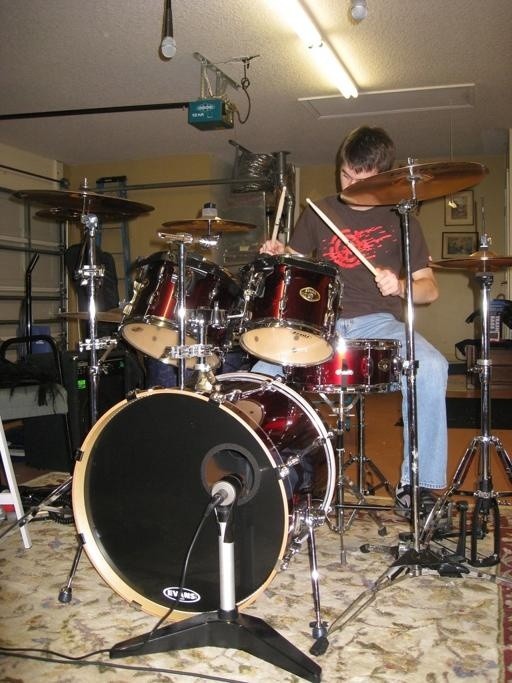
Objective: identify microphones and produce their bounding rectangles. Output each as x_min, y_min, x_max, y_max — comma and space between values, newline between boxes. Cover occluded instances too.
158, 1, 180, 63
348, 1, 373, 21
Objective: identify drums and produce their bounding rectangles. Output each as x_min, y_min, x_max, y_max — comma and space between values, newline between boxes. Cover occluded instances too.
72, 372, 337, 622
233, 252, 342, 368
286, 338, 403, 396
115, 249, 246, 369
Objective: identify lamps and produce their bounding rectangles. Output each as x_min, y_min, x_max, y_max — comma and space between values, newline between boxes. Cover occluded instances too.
278, 0, 359, 100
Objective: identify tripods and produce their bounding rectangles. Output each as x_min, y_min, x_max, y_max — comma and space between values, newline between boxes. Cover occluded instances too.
337, 395, 397, 533
309, 245, 512, 658
421, 275, 512, 548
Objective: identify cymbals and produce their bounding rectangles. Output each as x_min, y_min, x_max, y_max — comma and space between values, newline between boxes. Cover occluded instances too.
162, 215, 259, 236
31, 206, 141, 223
429, 247, 512, 271
57, 307, 125, 324
340, 160, 486, 204
10, 189, 155, 216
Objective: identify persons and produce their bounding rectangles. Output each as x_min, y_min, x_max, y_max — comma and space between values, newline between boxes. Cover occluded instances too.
260, 124, 454, 534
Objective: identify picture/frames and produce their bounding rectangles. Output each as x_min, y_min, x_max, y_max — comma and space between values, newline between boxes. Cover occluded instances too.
443, 190, 475, 225
441, 232, 479, 260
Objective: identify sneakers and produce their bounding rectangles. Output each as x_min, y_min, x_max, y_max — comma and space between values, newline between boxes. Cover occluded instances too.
395, 482, 448, 528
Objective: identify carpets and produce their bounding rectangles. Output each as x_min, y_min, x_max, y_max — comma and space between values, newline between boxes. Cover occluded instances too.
0, 472, 511, 682
